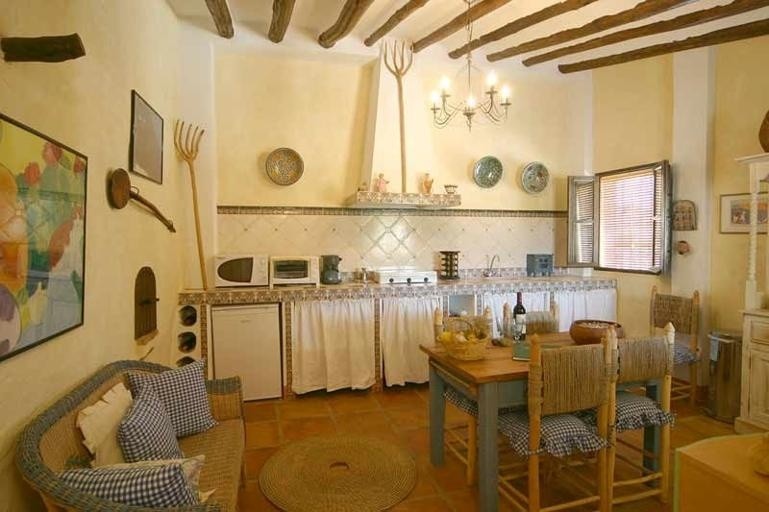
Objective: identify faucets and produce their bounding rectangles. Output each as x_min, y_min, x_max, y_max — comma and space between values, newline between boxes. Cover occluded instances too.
485, 254, 501, 277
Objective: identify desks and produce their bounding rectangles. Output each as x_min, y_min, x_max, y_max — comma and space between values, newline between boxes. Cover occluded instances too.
672, 429, 769, 511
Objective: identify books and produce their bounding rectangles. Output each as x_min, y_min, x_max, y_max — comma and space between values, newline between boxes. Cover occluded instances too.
511, 342, 562, 362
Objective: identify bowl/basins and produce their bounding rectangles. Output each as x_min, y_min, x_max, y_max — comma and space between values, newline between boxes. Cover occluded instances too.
570, 319, 624, 344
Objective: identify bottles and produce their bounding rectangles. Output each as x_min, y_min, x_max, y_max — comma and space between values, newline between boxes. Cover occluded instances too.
361, 268, 368, 281
512, 292, 527, 340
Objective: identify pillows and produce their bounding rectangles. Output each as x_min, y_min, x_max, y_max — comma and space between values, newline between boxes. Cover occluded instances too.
113, 379, 183, 462
121, 358, 221, 440
72, 380, 134, 466
54, 451, 217, 507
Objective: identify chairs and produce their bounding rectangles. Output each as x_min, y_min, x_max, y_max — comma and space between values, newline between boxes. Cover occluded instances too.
433, 307, 493, 486
563, 322, 675, 512
496, 332, 612, 512
500, 301, 559, 335
650, 285, 700, 414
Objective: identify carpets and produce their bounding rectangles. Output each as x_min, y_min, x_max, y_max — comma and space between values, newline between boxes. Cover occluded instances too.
258, 431, 419, 512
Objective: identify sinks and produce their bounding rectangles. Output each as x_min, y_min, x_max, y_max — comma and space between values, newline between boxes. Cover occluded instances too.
485, 277, 520, 281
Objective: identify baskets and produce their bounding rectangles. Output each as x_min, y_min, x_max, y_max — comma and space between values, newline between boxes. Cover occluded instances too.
435, 316, 491, 362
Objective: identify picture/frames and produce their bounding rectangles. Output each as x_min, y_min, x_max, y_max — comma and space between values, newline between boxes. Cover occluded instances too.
0, 114, 89, 365
718, 189, 769, 236
129, 88, 165, 186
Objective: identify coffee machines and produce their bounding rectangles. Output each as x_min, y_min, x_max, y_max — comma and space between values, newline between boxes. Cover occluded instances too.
321, 254, 341, 284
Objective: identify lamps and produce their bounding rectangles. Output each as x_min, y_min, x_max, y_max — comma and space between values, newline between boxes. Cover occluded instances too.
429, 0, 514, 132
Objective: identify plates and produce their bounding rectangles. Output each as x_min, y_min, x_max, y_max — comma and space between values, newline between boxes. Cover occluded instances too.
474, 156, 503, 188
522, 161, 551, 195
266, 148, 304, 186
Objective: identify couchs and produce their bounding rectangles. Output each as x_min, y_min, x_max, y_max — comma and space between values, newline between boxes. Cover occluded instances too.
14, 357, 246, 512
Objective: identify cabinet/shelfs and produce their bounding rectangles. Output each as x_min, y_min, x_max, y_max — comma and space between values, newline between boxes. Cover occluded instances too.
732, 308, 769, 435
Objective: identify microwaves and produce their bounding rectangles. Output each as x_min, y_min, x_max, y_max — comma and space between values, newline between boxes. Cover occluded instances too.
213, 253, 269, 288
269, 256, 321, 287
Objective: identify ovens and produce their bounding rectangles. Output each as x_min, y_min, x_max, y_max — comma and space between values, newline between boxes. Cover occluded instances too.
211, 302, 283, 401
